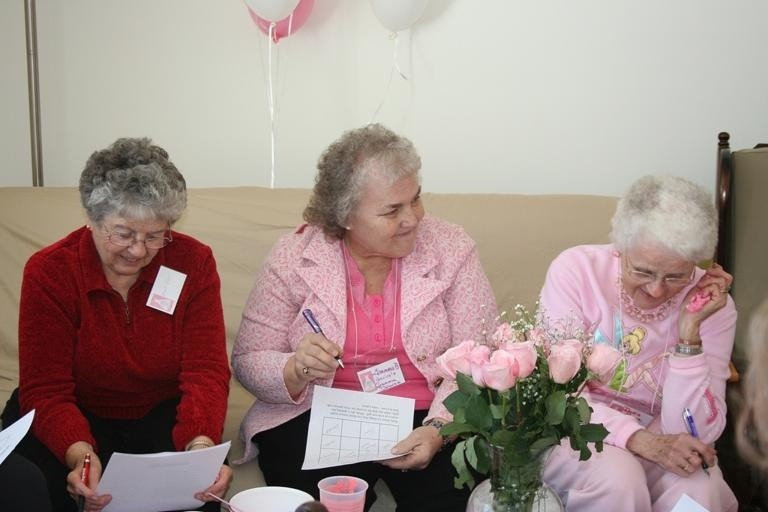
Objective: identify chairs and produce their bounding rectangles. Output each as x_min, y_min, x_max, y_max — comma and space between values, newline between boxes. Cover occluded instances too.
714, 131, 768, 497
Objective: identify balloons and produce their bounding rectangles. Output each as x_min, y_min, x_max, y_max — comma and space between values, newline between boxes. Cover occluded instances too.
370, 0, 427, 78
245, 0, 315, 188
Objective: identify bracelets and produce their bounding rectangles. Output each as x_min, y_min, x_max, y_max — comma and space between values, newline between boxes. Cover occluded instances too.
189, 441, 214, 446
679, 338, 703, 346
675, 346, 701, 354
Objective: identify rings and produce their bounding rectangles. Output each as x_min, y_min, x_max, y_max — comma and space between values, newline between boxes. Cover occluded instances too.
302, 367, 308, 377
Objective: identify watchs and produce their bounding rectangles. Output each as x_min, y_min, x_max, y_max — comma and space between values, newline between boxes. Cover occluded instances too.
426, 420, 451, 452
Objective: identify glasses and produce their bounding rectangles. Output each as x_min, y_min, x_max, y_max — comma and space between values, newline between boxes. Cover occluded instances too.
628, 250, 697, 290
100, 216, 174, 251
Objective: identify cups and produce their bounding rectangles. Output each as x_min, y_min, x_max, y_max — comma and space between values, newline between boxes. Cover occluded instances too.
317, 474, 370, 512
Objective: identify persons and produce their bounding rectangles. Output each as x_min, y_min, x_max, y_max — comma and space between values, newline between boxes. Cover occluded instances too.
231, 123, 498, 512
0, 136, 232, 512
536, 176, 739, 512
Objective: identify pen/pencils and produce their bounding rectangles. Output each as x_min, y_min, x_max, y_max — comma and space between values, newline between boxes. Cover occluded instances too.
682, 409, 710, 479
78, 453, 92, 512
302, 309, 344, 371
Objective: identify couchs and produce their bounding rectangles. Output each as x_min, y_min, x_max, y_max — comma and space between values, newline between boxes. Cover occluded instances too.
1, 186, 622, 512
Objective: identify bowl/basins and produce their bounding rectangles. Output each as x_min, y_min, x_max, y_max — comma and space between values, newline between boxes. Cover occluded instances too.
228, 485, 315, 512
